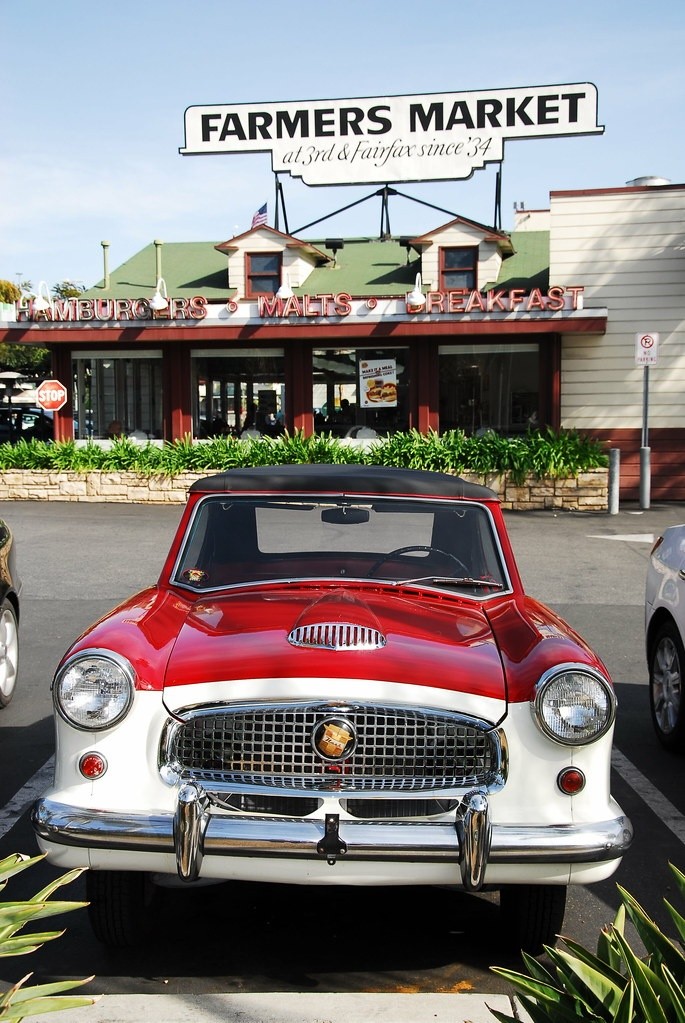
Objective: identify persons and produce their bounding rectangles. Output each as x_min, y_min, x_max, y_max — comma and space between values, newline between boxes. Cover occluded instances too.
199, 418, 238, 439
264, 408, 275, 436
99, 419, 127, 440
337, 399, 353, 425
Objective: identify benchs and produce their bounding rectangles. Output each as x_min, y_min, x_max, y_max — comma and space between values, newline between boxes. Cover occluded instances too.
218, 557, 452, 583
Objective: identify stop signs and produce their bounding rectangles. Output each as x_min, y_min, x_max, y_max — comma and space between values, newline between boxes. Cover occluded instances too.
35, 380, 70, 411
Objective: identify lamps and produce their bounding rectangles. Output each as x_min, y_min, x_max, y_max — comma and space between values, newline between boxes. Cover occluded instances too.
275, 271, 294, 300
148, 278, 168, 311
32, 280, 53, 311
407, 272, 425, 305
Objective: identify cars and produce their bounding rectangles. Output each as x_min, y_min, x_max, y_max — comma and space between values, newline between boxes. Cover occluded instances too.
0, 519, 24, 710
643, 524, 685, 753
32, 465, 636, 932
